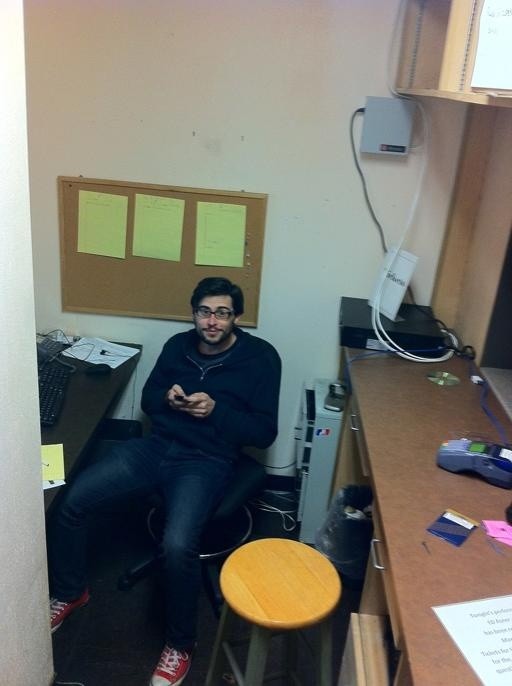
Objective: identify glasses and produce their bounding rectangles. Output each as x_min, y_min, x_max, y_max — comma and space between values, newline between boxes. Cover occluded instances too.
191, 306, 235, 320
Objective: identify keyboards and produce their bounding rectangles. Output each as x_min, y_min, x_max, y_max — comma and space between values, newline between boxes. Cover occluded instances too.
40, 367, 71, 427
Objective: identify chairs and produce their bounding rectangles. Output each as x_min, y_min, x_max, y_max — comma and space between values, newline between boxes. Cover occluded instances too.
115, 420, 278, 619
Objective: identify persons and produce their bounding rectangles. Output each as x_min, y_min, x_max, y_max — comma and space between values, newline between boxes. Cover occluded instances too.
45, 275, 281, 685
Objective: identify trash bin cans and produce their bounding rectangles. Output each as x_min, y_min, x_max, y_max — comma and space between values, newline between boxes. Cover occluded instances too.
315, 484, 375, 609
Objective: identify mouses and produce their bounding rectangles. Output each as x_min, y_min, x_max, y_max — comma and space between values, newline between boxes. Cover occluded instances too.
87, 363, 111, 374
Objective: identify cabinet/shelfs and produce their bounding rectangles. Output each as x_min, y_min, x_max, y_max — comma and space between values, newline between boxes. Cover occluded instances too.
393, 0, 510, 109
317, 331, 512, 686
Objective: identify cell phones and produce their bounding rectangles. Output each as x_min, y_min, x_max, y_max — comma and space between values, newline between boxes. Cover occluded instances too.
175, 395, 190, 403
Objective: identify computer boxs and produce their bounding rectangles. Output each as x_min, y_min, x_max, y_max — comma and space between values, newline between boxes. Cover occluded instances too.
80, 419, 143, 474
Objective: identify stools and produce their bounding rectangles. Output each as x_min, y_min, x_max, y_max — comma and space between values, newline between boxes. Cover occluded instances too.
205, 538, 343, 686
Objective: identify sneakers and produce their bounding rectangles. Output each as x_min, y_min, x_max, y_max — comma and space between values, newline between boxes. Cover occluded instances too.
49, 587, 90, 632
150, 641, 196, 685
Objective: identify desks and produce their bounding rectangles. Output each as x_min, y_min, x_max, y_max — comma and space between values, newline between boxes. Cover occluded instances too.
43, 337, 142, 513
